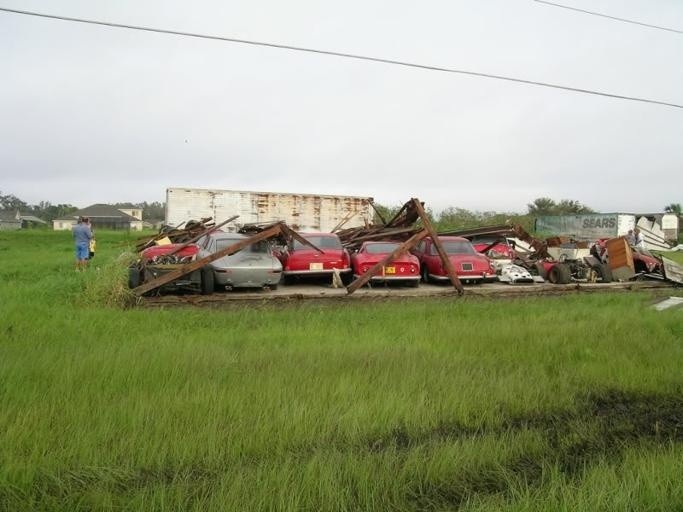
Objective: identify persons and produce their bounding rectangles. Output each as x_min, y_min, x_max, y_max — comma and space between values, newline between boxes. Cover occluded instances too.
624, 230, 635, 247
71, 216, 96, 271
86, 234, 95, 260
633, 227, 644, 249
